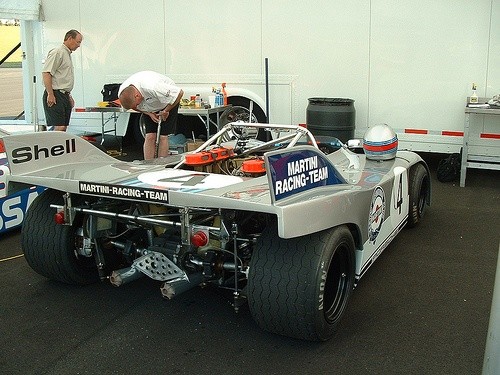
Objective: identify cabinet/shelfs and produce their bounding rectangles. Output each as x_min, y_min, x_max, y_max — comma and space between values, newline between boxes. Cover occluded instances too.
459, 101, 500, 188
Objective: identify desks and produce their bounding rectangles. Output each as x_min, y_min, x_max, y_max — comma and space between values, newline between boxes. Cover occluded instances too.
85, 101, 231, 154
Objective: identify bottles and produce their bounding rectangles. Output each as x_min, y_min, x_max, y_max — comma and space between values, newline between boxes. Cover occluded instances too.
195, 94, 201, 108
215, 91, 224, 107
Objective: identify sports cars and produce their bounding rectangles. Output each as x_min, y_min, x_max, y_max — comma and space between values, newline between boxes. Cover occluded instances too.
1, 118, 430, 342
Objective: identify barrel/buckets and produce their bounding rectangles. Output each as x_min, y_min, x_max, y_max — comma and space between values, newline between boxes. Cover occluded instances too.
306, 97, 355, 144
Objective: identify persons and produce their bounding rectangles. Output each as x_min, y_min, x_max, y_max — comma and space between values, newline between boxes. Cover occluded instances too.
118, 69, 184, 161
36, 29, 85, 132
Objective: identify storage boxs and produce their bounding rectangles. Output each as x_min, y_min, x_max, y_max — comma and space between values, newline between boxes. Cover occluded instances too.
184, 138, 206, 151
